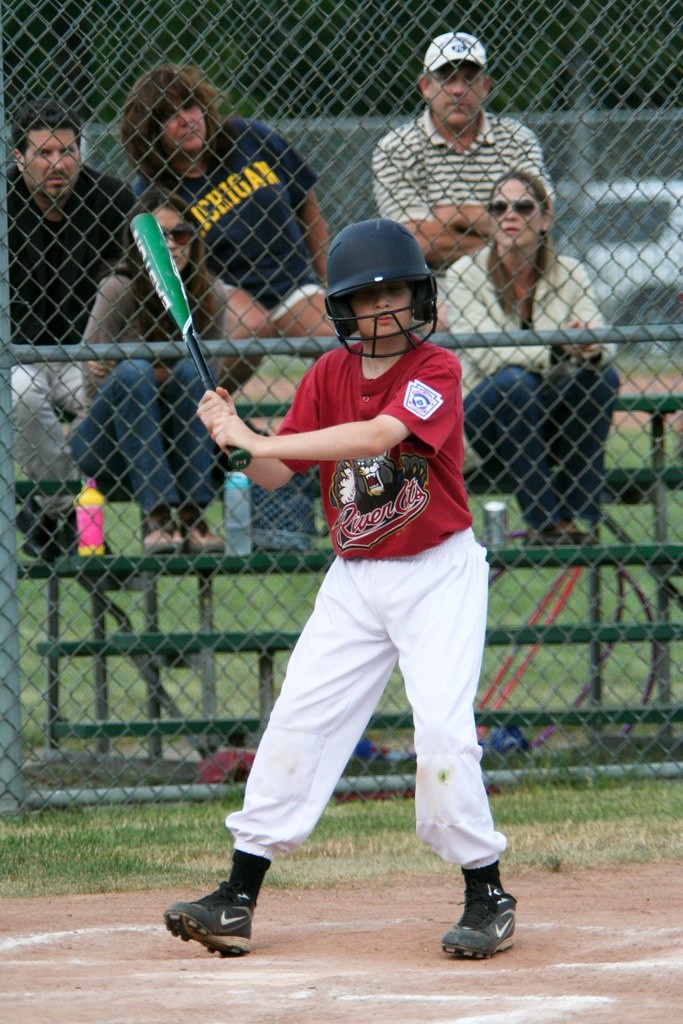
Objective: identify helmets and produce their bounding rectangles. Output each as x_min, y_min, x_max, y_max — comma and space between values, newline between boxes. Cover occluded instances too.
325, 220, 437, 341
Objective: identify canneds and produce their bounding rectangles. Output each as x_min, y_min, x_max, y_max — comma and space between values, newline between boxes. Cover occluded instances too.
483, 502, 506, 549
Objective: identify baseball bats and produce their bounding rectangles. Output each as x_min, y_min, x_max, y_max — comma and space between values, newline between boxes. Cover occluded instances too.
125, 211, 255, 475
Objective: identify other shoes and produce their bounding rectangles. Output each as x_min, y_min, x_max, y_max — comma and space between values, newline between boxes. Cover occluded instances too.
19, 501, 114, 562
180, 519, 225, 552
141, 519, 184, 552
526, 521, 599, 547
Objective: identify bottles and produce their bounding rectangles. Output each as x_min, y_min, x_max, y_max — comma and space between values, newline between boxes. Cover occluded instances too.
73, 478, 106, 556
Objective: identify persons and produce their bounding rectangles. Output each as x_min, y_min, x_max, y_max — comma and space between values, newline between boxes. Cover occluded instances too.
372, 32, 556, 293
443, 169, 620, 545
122, 64, 340, 395
67, 190, 228, 554
164, 218, 518, 961
5, 100, 135, 561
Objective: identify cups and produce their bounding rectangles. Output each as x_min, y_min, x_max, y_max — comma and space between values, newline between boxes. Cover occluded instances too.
223, 471, 251, 557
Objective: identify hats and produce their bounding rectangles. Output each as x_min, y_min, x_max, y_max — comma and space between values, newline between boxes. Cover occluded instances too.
423, 31, 488, 72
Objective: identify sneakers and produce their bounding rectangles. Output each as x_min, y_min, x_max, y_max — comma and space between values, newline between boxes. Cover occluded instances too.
164, 879, 257, 957
440, 879, 517, 958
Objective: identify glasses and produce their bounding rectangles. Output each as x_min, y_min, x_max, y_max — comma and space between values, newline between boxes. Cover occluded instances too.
487, 199, 538, 216
161, 222, 192, 245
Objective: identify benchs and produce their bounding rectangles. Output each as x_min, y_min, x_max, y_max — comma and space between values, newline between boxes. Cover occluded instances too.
0, 390, 683, 740
